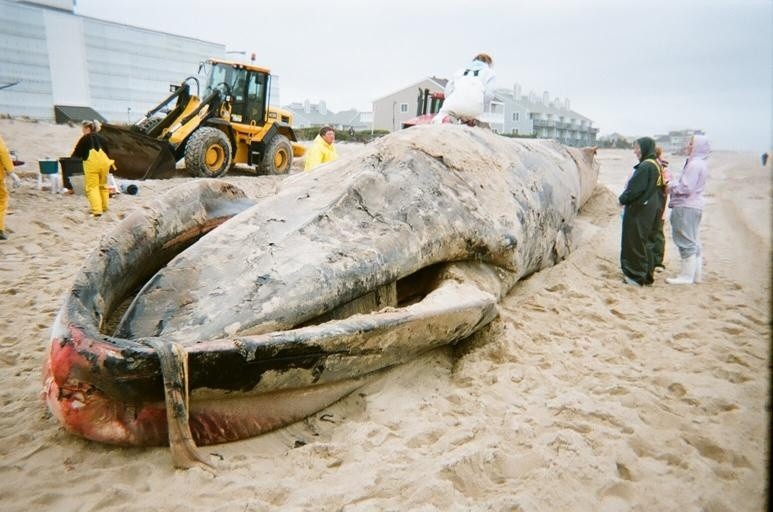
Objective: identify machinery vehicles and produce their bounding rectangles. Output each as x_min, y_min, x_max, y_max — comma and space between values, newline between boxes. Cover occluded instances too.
400, 86, 474, 128
71, 58, 304, 181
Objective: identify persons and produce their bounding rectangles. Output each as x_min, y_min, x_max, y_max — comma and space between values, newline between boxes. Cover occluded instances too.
619, 134, 713, 286
70, 120, 111, 218
304, 126, 338, 171
0, 135, 14, 240
432, 53, 503, 125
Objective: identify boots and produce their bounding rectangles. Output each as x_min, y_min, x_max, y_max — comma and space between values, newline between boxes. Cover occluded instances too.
664, 253, 703, 286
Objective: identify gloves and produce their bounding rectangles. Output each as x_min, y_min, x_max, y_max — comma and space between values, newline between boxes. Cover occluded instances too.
9, 172, 21, 188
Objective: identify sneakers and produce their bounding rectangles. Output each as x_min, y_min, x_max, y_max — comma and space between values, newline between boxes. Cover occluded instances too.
0, 230, 9, 239
448, 116, 481, 126
